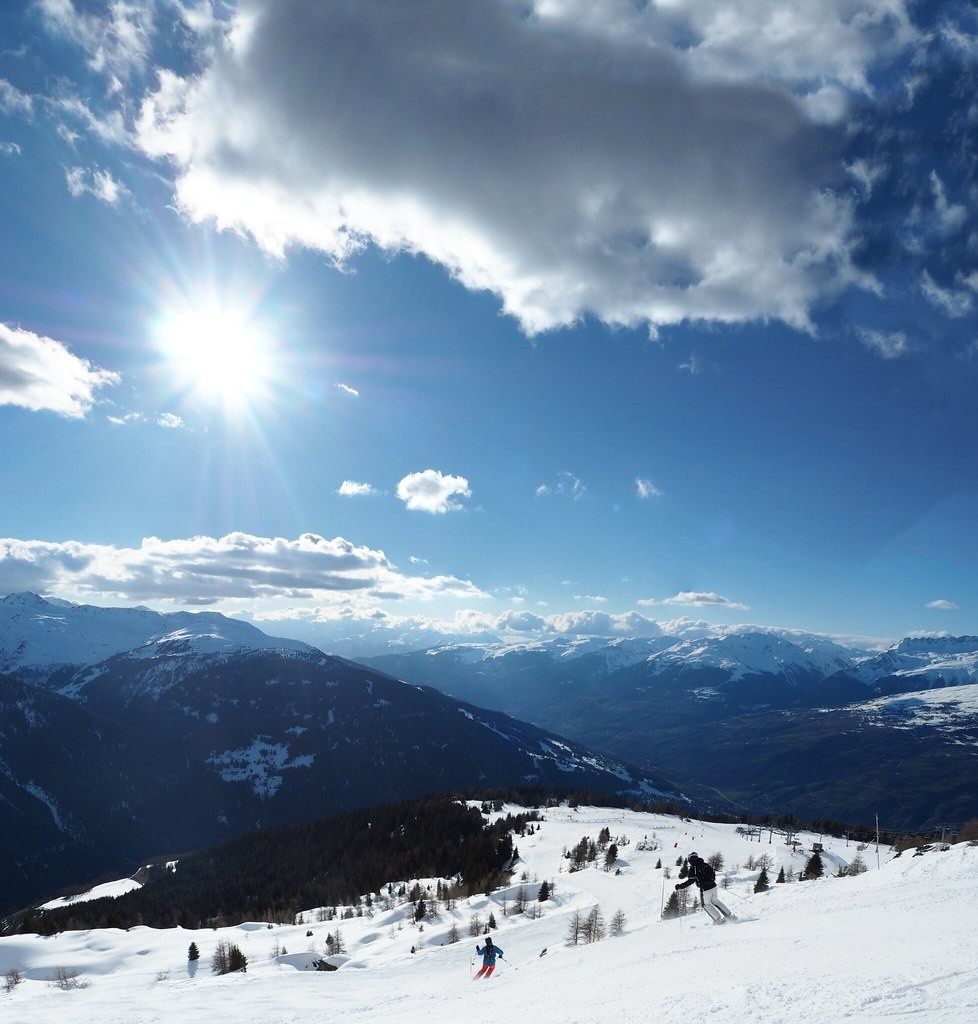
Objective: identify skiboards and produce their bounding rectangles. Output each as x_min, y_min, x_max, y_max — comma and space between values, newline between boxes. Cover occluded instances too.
692, 918, 760, 926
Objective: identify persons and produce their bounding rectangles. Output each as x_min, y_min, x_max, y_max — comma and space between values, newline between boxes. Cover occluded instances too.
675, 855, 732, 926
473, 937, 504, 980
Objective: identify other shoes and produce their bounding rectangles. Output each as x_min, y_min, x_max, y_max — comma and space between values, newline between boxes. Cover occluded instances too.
712, 918, 722, 925
723, 915, 732, 921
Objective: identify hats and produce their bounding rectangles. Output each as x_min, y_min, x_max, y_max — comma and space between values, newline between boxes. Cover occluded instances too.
688, 851, 698, 863
485, 937, 492, 943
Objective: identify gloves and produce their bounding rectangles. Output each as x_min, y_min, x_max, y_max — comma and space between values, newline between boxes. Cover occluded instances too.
675, 884, 680, 890
476, 945, 479, 949
498, 955, 502, 958
